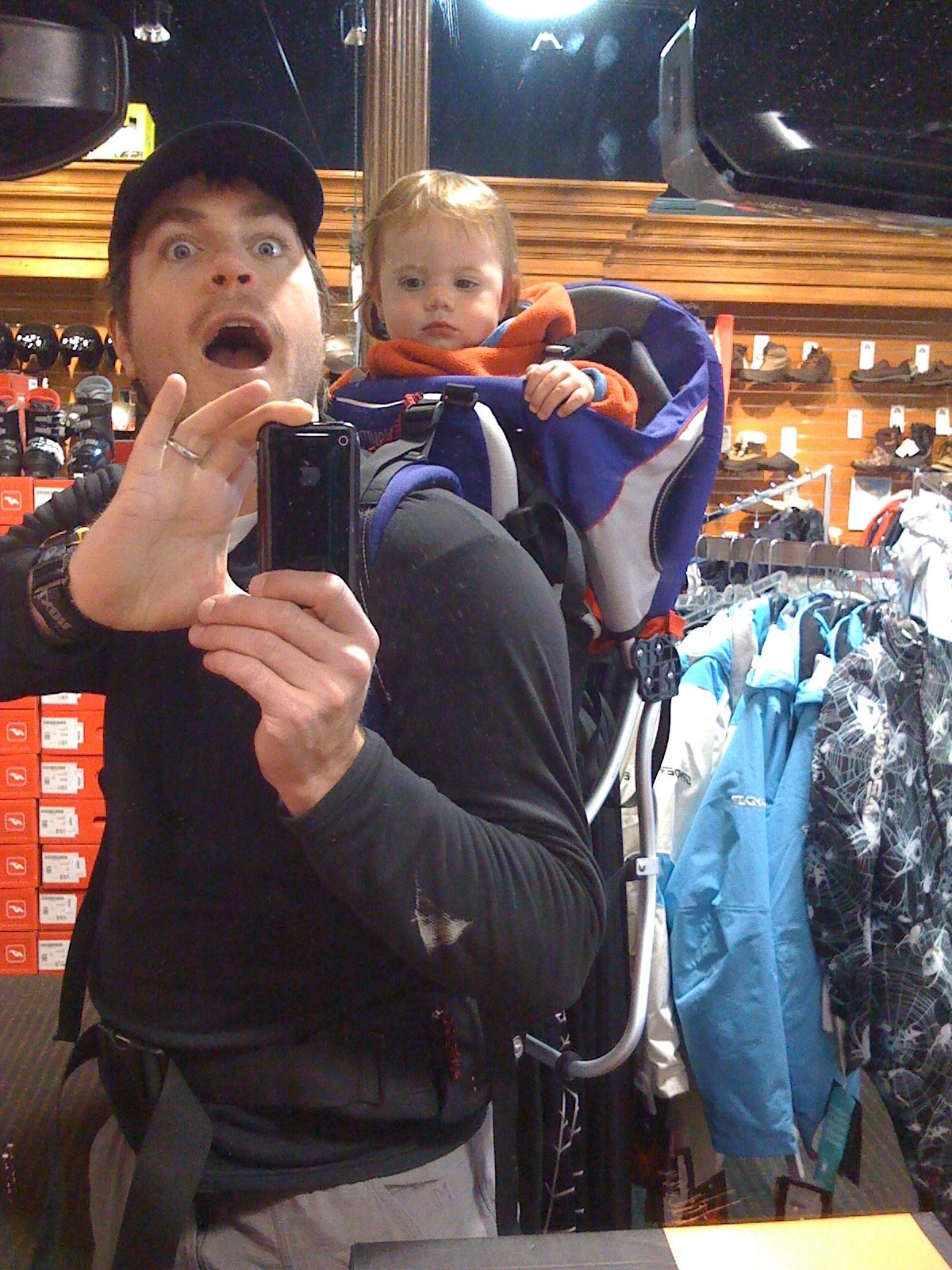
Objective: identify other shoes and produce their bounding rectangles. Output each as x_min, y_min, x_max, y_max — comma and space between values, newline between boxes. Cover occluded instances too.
851, 425, 904, 471
783, 347, 834, 384
891, 421, 937, 472
738, 342, 790, 384
930, 438, 952, 472
912, 361, 952, 386
850, 359, 912, 384
717, 440, 767, 470
755, 452, 800, 472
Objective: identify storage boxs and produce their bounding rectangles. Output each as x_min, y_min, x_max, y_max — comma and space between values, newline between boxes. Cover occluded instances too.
0, 694, 106, 977
0, 476, 68, 538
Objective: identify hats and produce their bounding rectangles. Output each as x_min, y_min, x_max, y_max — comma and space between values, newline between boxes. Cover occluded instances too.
61, 324, 104, 364
111, 117, 325, 255
12, 323, 59, 369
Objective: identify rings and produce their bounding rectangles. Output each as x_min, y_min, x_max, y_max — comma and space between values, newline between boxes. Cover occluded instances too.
167, 437, 201, 463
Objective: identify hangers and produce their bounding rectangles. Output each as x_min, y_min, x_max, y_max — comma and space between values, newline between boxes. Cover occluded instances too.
675, 532, 920, 632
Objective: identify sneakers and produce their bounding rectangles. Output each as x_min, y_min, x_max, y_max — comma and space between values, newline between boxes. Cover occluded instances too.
0, 386, 25, 477
22, 386, 68, 480
64, 374, 116, 479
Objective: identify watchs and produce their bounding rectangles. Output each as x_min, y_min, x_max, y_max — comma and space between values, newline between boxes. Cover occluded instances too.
31, 523, 107, 643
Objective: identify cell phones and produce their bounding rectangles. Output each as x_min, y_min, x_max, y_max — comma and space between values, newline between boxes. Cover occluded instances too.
256, 420, 358, 613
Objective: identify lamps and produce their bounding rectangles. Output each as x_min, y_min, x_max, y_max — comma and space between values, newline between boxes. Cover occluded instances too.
131, 0, 175, 43
531, 21, 568, 54
338, 2, 366, 46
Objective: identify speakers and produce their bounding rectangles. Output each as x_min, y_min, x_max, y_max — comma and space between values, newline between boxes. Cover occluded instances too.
657, 2, 952, 235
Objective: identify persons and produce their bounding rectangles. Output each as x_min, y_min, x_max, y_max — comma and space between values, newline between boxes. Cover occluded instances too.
1, 122, 610, 1270
329, 167, 640, 641
